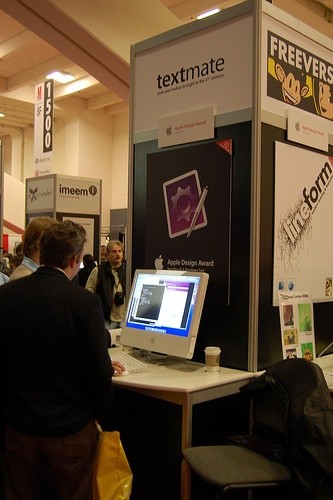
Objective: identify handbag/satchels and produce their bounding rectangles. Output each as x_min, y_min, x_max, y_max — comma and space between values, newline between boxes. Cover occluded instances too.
89, 418, 133, 500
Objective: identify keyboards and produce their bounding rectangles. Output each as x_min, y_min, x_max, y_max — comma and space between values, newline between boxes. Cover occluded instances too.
108, 346, 147, 375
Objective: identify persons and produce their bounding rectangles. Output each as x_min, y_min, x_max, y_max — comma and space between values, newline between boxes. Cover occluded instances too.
86, 240, 126, 329
78, 254, 96, 287
3, 242, 23, 277
10, 217, 58, 280
0, 221, 126, 500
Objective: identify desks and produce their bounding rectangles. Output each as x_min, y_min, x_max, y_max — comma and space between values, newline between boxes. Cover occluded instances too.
111, 347, 259, 500
254, 353, 333, 391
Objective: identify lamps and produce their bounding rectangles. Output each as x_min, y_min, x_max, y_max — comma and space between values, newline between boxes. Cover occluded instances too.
47, 70, 76, 84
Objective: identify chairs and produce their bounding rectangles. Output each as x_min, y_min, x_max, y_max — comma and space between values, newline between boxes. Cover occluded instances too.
184, 359, 333, 500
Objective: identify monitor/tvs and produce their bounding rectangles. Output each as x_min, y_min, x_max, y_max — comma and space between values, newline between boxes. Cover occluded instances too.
118, 269, 210, 360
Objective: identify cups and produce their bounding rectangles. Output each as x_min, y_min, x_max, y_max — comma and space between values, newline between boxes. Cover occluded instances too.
204, 346, 221, 375
